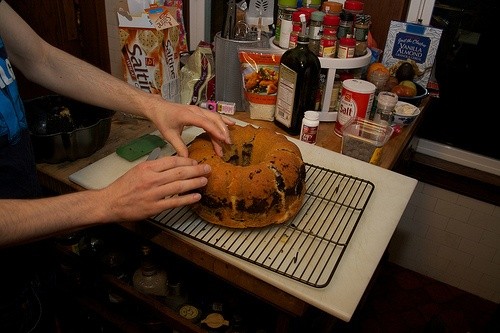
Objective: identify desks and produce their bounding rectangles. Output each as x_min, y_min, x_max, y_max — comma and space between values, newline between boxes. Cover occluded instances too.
35, 94, 432, 333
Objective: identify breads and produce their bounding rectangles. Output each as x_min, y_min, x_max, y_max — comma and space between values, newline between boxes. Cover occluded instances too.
181, 124, 306, 228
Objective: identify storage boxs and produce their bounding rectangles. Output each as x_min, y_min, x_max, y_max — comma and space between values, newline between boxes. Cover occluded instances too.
117, 4, 182, 103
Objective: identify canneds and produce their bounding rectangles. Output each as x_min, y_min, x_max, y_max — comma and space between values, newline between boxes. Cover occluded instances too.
334, 78, 376, 137
279, 0, 372, 59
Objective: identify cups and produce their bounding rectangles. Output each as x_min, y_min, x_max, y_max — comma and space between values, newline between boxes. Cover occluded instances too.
213, 33, 268, 111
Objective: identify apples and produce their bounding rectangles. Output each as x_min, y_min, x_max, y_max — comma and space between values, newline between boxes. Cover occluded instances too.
367, 63, 416, 96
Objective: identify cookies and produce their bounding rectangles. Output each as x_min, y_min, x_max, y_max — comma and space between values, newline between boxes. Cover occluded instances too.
120, 27, 179, 90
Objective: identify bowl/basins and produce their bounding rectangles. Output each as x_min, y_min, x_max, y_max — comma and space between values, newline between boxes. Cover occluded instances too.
375, 101, 421, 126
23, 96, 115, 163
374, 81, 428, 105
338, 116, 394, 165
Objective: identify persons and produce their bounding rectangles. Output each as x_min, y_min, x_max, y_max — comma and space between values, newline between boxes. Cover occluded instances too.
0, 0, 236, 249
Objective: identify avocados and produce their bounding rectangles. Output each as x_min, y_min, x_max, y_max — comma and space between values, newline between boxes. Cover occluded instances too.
396, 62, 415, 85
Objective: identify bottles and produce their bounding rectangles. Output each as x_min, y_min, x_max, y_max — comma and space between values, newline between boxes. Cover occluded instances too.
274, 32, 321, 136
370, 91, 398, 123
300, 111, 321, 144
271, 0, 372, 59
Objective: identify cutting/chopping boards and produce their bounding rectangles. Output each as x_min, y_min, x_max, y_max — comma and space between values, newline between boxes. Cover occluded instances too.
67, 113, 419, 322
